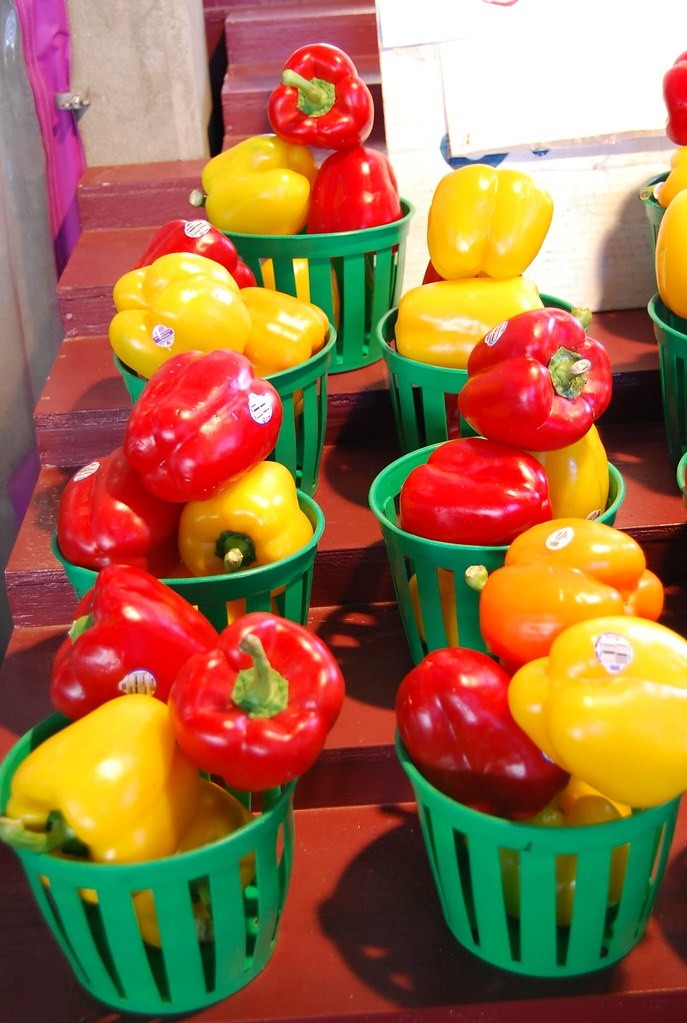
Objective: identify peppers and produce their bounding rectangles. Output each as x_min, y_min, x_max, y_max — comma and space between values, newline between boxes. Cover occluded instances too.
0, 44, 687, 949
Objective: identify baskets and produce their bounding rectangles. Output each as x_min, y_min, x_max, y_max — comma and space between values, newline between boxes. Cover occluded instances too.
113, 322, 338, 498
394, 710, 683, 976
0, 709, 300, 1014
368, 436, 625, 675
206, 197, 415, 374
375, 293, 588, 455
648, 288, 687, 468
642, 171, 687, 292
50, 487, 325, 636
